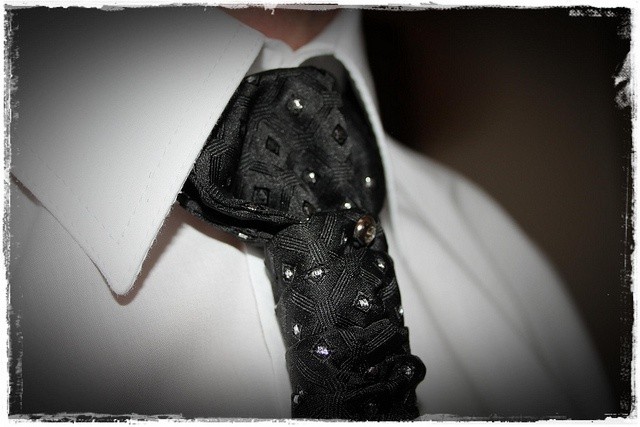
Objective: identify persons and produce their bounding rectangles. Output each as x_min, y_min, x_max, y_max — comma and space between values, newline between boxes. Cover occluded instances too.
10, 10, 614, 418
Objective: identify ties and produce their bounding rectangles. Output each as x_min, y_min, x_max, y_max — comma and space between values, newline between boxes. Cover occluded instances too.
176, 55, 426, 419
